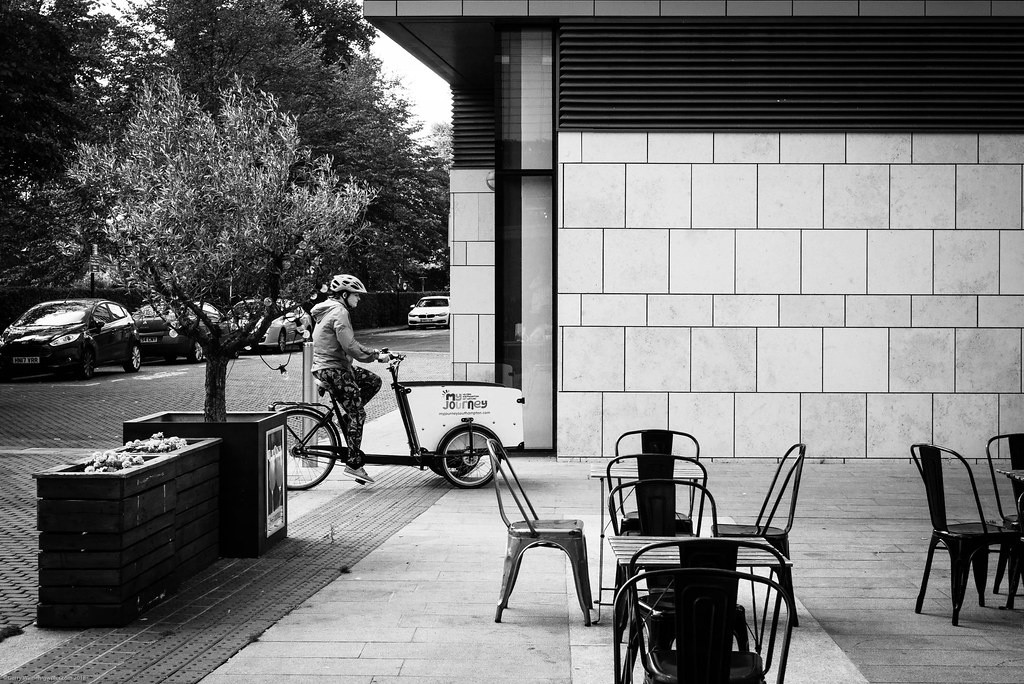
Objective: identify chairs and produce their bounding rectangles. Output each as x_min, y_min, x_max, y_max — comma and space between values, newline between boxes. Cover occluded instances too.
910, 432, 1024, 625
486, 429, 807, 684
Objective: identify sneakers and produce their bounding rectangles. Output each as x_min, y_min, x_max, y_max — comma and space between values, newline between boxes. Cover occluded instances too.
334, 413, 348, 428
343, 466, 374, 484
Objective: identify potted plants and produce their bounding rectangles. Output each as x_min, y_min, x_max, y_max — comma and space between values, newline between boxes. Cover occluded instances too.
63, 74, 379, 560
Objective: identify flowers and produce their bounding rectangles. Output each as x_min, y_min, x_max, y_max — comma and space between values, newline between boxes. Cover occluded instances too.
84, 432, 188, 472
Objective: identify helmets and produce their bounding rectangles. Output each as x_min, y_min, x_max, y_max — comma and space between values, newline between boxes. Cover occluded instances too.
330, 274, 368, 293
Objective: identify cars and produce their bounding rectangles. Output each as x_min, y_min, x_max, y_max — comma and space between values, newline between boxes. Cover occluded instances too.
0, 297, 143, 381
407, 295, 451, 330
226, 297, 314, 354
131, 301, 225, 364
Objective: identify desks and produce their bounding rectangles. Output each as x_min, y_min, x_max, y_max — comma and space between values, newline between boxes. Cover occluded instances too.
994, 469, 1024, 596
589, 468, 708, 603
608, 534, 795, 654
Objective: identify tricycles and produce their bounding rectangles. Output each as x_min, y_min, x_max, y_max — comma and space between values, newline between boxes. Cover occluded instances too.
268, 346, 527, 491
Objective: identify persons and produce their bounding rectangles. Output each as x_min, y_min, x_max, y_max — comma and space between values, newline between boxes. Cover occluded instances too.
310, 275, 391, 484
